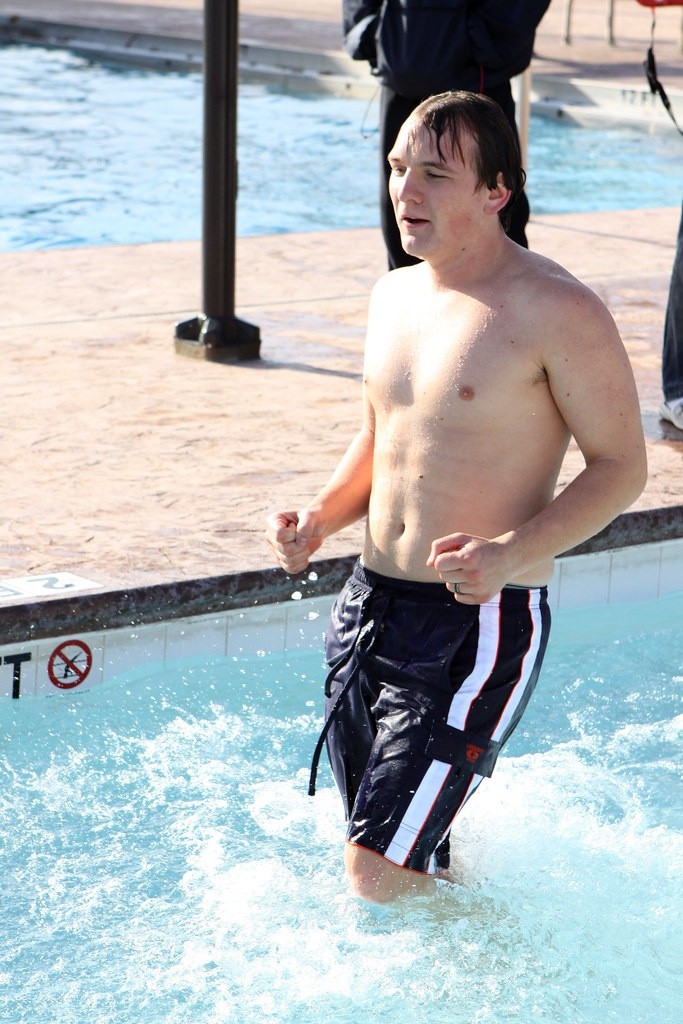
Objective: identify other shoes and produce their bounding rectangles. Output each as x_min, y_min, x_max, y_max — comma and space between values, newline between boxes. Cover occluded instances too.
659, 402, 683, 430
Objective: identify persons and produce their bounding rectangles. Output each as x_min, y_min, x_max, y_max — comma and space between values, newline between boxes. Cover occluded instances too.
344, 0, 550, 271
660, 199, 683, 433
267, 92, 647, 905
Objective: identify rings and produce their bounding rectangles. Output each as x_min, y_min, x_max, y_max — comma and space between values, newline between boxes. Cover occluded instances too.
454, 583, 463, 594
